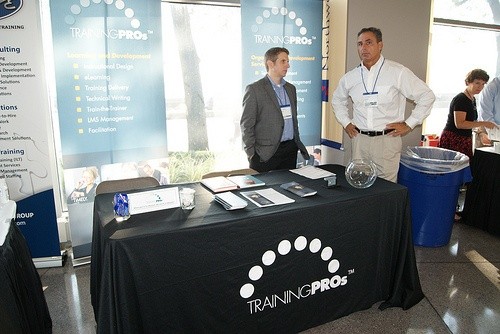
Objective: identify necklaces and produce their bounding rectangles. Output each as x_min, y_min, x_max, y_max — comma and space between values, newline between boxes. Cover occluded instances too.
465, 88, 474, 102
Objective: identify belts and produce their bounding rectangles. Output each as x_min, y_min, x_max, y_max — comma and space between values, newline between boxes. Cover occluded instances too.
354, 126, 394, 137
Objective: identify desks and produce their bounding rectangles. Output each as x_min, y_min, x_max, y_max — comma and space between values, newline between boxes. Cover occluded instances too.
457, 144, 500, 237
0, 199, 54, 334
91, 164, 429, 334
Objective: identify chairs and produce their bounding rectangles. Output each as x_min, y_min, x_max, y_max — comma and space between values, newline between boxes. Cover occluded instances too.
95, 177, 160, 195
203, 169, 258, 180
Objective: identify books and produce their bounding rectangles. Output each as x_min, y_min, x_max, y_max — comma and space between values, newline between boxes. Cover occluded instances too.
227, 175, 265, 189
213, 191, 249, 211
200, 176, 237, 193
289, 164, 336, 180
240, 187, 296, 208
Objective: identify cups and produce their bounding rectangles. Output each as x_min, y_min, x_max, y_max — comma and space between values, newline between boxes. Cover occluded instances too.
179, 188, 196, 210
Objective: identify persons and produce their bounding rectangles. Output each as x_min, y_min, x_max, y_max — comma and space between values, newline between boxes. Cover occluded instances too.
312, 148, 321, 165
439, 68, 500, 225
474, 74, 500, 149
332, 27, 436, 184
239, 47, 310, 174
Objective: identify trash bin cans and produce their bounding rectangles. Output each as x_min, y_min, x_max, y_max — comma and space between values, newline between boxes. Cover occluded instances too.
397, 145, 473, 248
